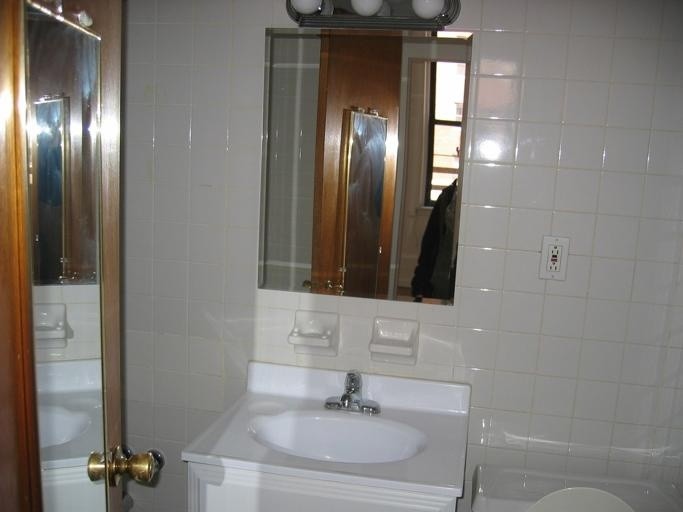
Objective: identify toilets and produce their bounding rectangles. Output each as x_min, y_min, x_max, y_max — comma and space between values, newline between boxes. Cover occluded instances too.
470, 467, 680, 512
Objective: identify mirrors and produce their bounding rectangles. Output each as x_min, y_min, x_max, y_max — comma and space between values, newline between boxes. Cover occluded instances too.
0, 0, 122, 511
257, 27, 473, 306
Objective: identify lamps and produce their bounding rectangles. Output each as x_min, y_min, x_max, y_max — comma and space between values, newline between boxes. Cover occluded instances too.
285, 0, 460, 31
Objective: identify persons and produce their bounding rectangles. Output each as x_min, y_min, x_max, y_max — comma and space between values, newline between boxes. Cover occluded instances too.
412, 179, 457, 301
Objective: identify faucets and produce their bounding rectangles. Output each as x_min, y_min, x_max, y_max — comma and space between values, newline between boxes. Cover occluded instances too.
324, 371, 379, 414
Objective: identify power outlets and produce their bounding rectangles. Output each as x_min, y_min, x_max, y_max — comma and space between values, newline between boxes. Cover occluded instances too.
540, 236, 569, 280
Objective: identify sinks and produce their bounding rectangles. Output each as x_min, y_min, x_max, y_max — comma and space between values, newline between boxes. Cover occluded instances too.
180, 394, 469, 498
35, 358, 104, 469
